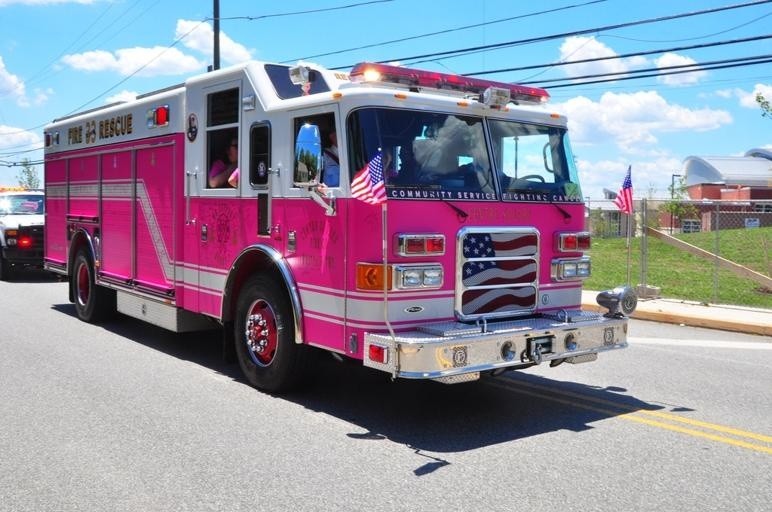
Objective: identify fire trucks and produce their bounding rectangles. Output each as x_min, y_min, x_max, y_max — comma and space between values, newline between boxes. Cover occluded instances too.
38, 56, 640, 398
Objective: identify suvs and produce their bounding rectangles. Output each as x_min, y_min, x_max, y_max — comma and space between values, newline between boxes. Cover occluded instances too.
0, 183, 46, 277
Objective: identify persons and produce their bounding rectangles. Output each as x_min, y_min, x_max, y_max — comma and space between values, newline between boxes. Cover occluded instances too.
321, 126, 339, 188
382, 149, 397, 177
228, 168, 239, 188
208, 137, 238, 188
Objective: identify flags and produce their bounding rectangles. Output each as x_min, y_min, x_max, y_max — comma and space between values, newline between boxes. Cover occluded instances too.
611, 165, 634, 215
350, 153, 389, 206
